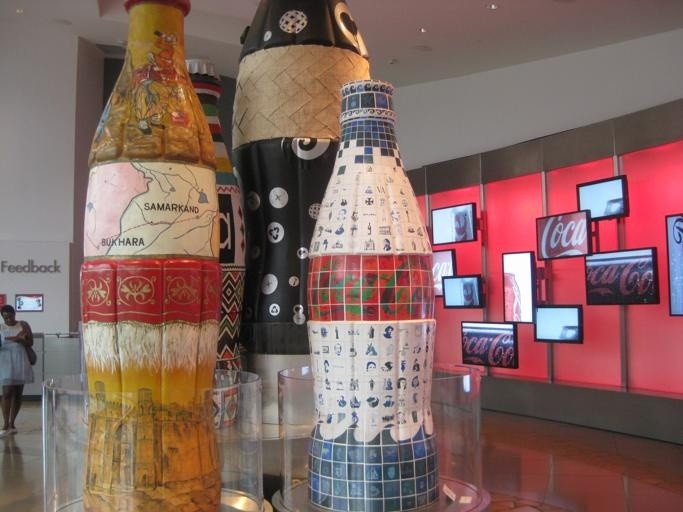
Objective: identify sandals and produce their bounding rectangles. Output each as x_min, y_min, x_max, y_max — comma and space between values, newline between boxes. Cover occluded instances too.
0, 428, 17, 437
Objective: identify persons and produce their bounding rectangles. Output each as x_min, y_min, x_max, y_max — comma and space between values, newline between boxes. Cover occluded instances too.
0, 304, 36, 437
0, 436, 37, 511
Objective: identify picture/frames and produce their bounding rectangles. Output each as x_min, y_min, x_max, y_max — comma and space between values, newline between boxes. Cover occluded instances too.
432, 202, 477, 245
15, 294, 43, 312
432, 249, 457, 297
535, 304, 584, 344
502, 251, 536, 324
461, 320, 518, 369
665, 213, 683, 316
584, 246, 660, 304
441, 274, 483, 309
576, 175, 629, 220
535, 209, 592, 260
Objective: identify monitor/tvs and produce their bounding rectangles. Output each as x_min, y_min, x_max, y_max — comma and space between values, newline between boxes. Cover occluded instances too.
15, 293, 44, 313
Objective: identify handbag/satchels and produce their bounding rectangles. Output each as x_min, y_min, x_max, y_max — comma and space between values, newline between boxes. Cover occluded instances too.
24, 346, 36, 365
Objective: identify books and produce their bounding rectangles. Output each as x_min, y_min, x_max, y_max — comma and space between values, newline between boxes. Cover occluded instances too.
4, 330, 27, 343
